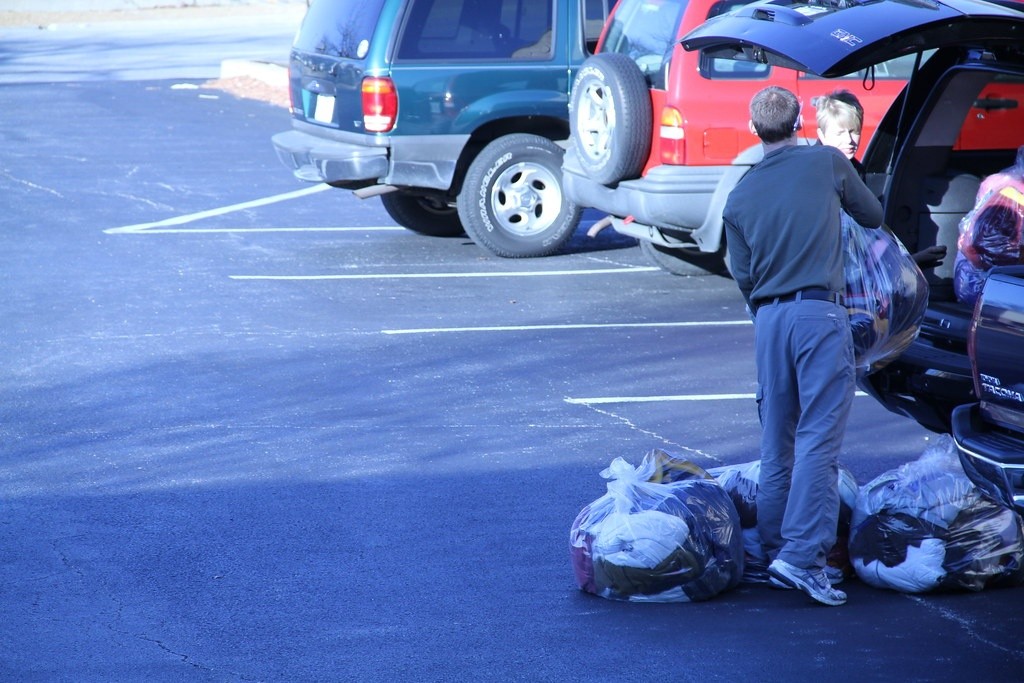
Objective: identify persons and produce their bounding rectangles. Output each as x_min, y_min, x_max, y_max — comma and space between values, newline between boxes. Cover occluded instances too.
813, 90, 866, 184
722, 86, 886, 608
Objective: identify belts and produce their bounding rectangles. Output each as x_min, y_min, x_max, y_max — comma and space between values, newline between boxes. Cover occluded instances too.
758, 288, 845, 306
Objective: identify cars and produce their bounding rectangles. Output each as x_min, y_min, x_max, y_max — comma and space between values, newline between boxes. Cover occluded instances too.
271, 0, 621, 260
678, 0, 1024, 439
950, 265, 1024, 516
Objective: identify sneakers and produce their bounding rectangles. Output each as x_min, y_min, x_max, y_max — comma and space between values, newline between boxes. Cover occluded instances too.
767, 559, 847, 606
769, 563, 843, 588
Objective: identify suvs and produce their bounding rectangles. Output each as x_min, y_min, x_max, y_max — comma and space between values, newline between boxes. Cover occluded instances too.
561, 0, 938, 277
